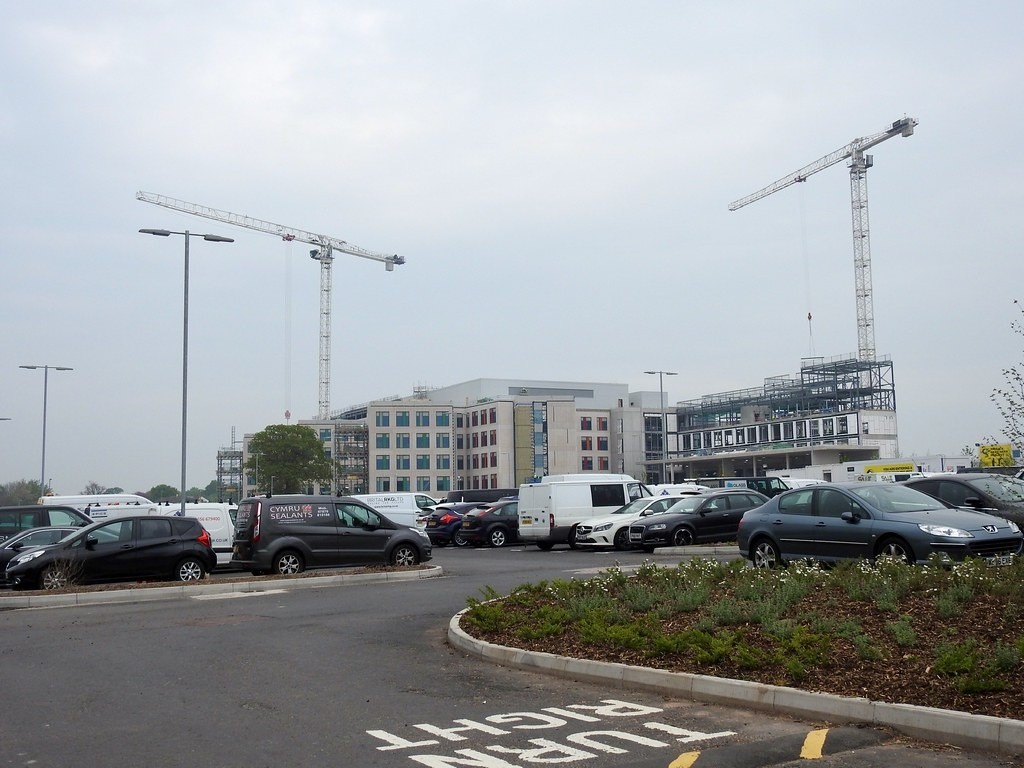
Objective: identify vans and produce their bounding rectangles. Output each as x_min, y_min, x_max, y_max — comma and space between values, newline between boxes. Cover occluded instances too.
339, 492, 439, 536
516, 480, 658, 551
854, 472, 925, 483
542, 474, 635, 483
629, 483, 711, 498
36, 494, 153, 525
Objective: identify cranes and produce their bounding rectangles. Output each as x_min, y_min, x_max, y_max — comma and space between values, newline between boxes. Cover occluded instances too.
132, 190, 406, 420
729, 114, 920, 386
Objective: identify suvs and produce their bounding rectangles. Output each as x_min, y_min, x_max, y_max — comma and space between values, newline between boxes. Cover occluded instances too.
0, 506, 122, 555
229, 494, 433, 576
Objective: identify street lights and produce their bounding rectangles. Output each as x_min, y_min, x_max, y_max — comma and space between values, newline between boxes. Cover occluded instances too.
643, 370, 679, 484
19, 364, 75, 495
137, 227, 234, 517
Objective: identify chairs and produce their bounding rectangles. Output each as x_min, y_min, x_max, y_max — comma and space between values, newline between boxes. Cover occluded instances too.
859, 496, 879, 518
120, 526, 131, 541
822, 494, 851, 515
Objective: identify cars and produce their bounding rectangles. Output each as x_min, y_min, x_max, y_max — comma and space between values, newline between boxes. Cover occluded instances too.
900, 473, 1024, 527
628, 486, 773, 552
734, 481, 1023, 573
576, 494, 717, 552
460, 501, 519, 548
1, 526, 120, 580
412, 500, 488, 546
5, 516, 220, 591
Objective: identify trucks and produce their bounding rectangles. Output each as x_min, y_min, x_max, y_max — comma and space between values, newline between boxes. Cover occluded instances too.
80, 501, 242, 554
